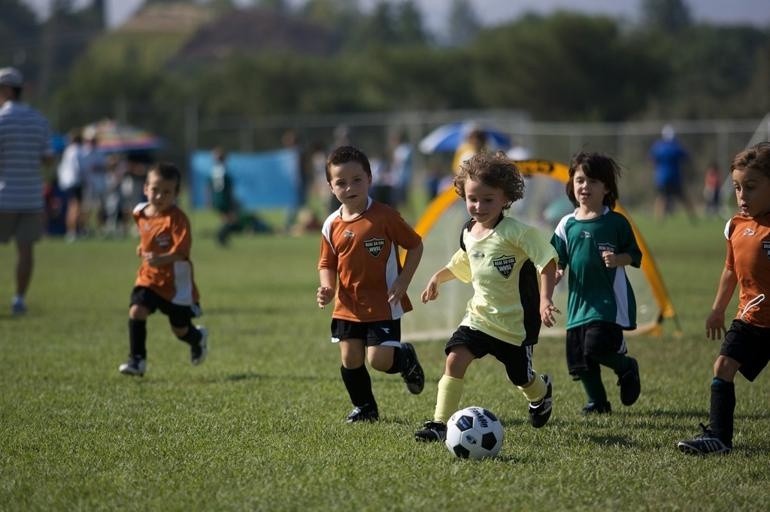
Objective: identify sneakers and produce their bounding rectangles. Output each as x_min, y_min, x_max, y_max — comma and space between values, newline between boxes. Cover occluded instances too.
119, 356, 145, 376
415, 421, 447, 442
191, 326, 207, 364
14, 298, 27, 313
346, 404, 378, 422
678, 435, 732, 457
617, 357, 641, 406
399, 343, 424, 394
529, 376, 552, 428
581, 403, 612, 414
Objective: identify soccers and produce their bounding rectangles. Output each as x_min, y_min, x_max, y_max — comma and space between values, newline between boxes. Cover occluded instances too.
442, 406, 505, 459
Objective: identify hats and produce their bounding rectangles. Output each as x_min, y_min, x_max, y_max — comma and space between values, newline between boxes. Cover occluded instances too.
1, 69, 25, 87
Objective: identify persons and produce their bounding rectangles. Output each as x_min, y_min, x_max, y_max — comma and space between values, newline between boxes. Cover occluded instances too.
44, 122, 745, 253
313, 144, 427, 423
115, 161, 210, 380
0, 65, 55, 313
548, 150, 645, 414
411, 151, 562, 445
674, 142, 770, 458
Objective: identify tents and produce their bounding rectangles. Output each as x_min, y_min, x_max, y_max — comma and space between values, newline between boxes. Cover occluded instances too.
396, 157, 686, 350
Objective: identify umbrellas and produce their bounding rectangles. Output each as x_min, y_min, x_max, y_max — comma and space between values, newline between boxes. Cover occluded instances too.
419, 115, 517, 155
92, 116, 174, 155
